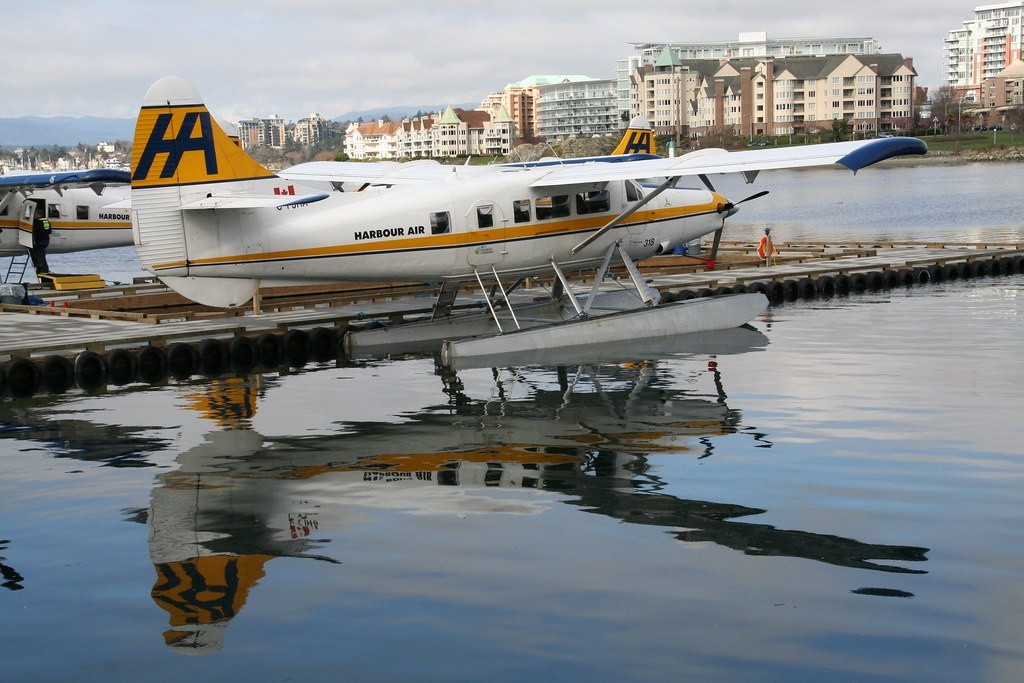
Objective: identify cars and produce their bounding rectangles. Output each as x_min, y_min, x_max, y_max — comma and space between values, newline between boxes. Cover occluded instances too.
974, 126, 987, 132
747, 141, 755, 147
879, 132, 894, 138
758, 141, 769, 146
989, 125, 1002, 131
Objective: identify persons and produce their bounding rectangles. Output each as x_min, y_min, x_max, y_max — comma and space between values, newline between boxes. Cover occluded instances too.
49, 205, 61, 218
30, 209, 50, 283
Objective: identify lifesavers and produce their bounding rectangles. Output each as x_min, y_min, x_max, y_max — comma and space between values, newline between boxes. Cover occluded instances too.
393, 319, 410, 326
257, 333, 284, 370
310, 327, 336, 363
335, 324, 359, 360
757, 236, 774, 260
196, 338, 229, 378
38, 354, 75, 395
167, 342, 199, 380
136, 345, 168, 385
363, 322, 388, 358
74, 350, 107, 391
108, 347, 137, 386
697, 254, 1024, 305
5, 356, 42, 398
676, 289, 697, 301
657, 292, 677, 304
228, 336, 258, 374
281, 329, 312, 367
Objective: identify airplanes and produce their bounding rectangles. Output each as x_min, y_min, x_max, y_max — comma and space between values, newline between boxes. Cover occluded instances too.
119, 365, 747, 655
1, 103, 927, 375
